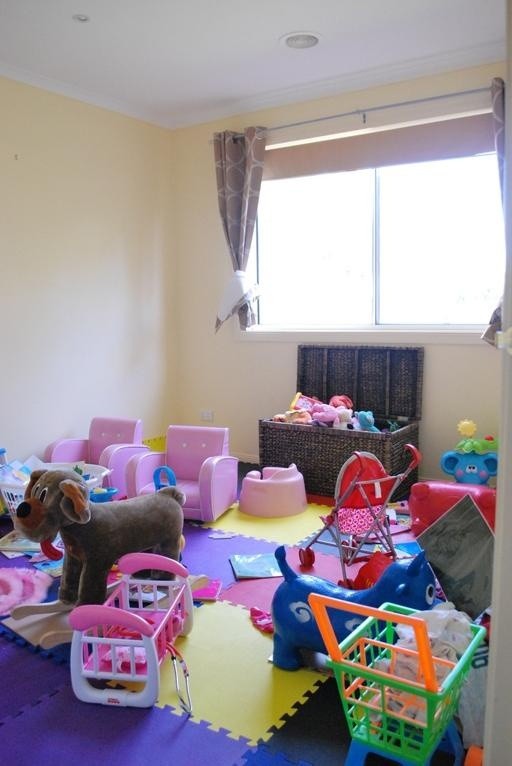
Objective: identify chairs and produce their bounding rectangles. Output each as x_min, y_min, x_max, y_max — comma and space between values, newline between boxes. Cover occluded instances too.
47, 415, 239, 526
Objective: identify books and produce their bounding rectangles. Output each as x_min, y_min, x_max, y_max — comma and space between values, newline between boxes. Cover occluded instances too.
230, 554, 283, 577
193, 579, 222, 599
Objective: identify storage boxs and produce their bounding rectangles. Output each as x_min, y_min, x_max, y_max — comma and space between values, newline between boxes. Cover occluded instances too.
258, 341, 425, 505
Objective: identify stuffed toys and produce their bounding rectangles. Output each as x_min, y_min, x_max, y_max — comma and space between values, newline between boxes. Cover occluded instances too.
273, 390, 400, 434
